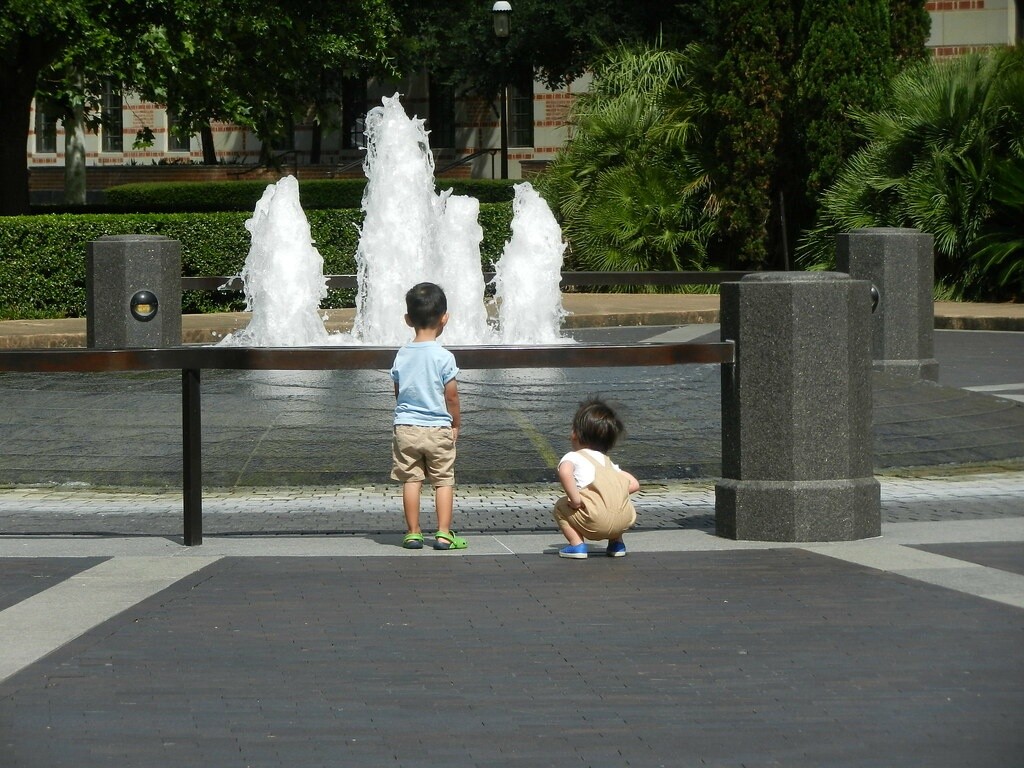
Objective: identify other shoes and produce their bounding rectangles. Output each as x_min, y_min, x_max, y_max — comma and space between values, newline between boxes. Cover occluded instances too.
605, 542, 627, 556
558, 543, 589, 559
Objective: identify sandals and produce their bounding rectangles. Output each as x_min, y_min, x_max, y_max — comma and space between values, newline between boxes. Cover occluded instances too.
433, 529, 469, 550
401, 531, 425, 548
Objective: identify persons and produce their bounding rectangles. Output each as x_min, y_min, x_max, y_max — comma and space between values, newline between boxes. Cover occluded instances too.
389, 282, 469, 550
553, 399, 640, 559
